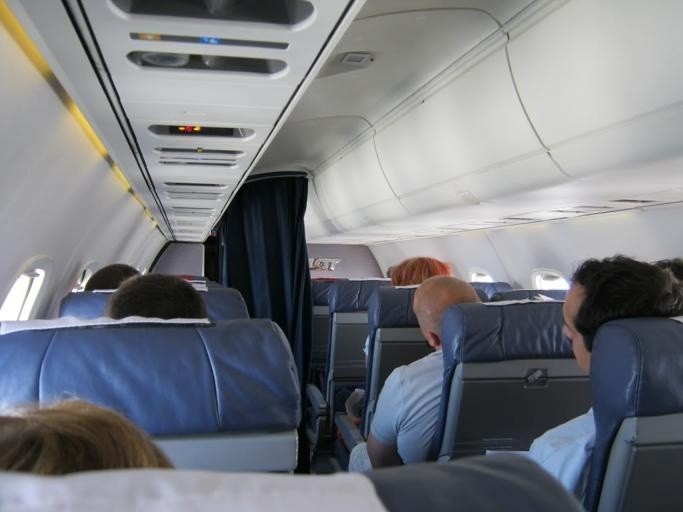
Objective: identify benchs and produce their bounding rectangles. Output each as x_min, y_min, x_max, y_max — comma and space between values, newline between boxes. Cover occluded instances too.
0, 278, 683, 511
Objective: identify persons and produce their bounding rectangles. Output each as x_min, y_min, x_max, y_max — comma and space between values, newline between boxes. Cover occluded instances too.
106, 272, 206, 321
84, 263, 143, 293
345, 274, 488, 475
0, 397, 175, 478
332, 258, 452, 433
511, 252, 682, 499
